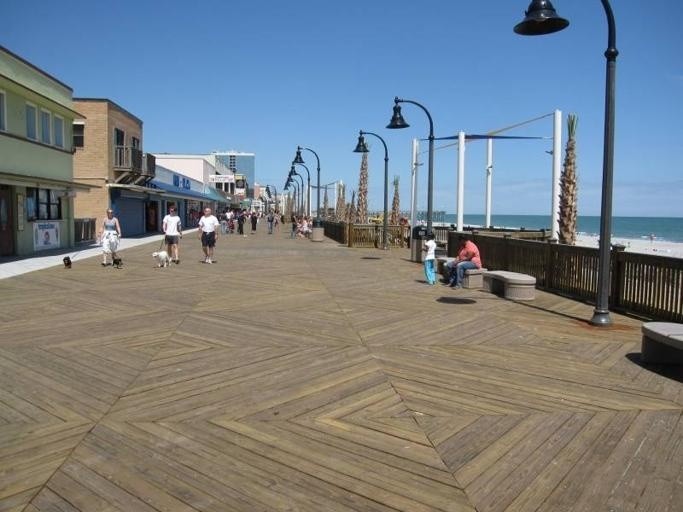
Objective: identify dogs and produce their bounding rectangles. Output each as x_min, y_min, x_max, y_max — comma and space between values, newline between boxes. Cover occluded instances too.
112, 257, 123, 269
152, 251, 172, 268
62, 256, 72, 268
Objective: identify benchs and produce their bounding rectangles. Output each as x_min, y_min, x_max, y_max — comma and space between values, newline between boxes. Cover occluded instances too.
639, 321, 682, 364
437, 256, 536, 301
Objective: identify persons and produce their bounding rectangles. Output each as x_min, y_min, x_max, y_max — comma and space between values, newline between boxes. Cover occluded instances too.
420, 233, 436, 285
98, 208, 123, 267
162, 206, 183, 265
444, 235, 482, 290
42, 232, 51, 246
190, 206, 314, 264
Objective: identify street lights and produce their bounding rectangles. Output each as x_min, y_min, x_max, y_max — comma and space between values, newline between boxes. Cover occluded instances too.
284, 146, 325, 242
513, 0, 619, 324
352, 130, 388, 249
386, 97, 434, 239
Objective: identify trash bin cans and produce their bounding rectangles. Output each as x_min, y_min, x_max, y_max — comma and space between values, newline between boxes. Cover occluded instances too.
412, 226, 432, 263
312, 221, 324, 241
